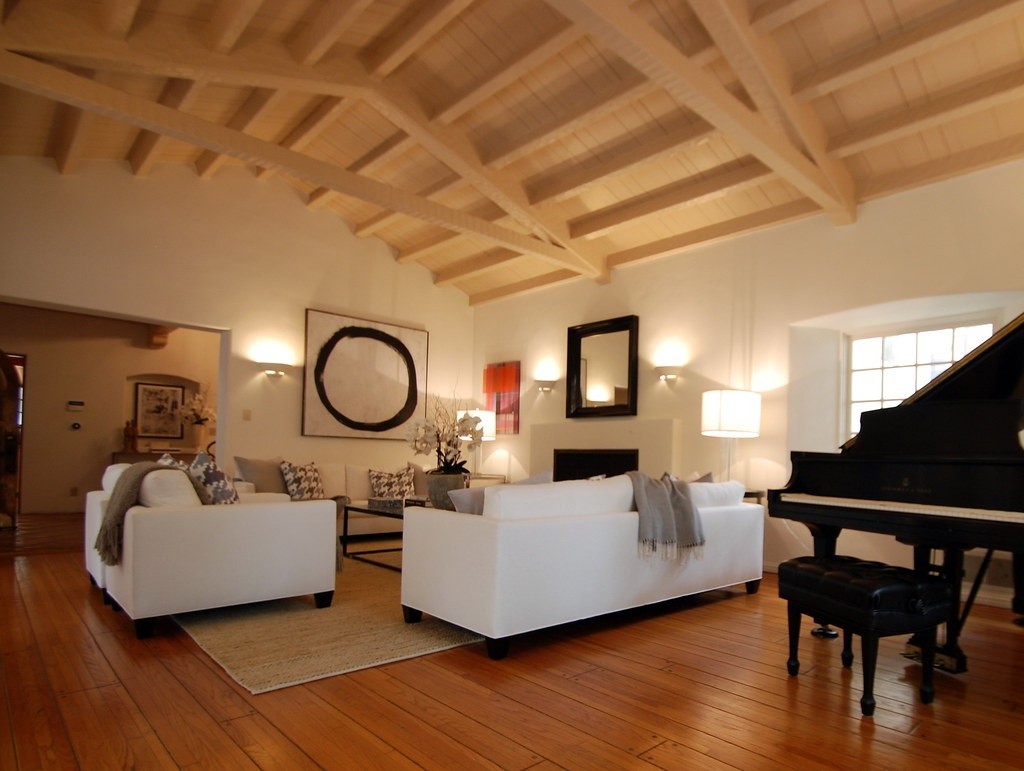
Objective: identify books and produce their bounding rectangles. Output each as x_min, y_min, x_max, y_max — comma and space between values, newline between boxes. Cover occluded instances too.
405, 499, 432, 507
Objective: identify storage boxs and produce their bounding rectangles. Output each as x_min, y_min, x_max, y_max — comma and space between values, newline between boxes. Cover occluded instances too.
405, 498, 429, 507
367, 499, 404, 511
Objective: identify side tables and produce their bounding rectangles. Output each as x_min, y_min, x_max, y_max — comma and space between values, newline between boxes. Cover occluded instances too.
470, 473, 506, 487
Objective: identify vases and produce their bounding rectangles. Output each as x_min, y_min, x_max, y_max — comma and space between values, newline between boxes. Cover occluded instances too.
425, 471, 470, 510
192, 426, 207, 447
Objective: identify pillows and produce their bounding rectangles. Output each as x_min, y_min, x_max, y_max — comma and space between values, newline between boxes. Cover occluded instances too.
157, 453, 749, 516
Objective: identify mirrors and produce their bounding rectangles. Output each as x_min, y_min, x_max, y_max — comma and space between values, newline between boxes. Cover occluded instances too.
565, 315, 641, 418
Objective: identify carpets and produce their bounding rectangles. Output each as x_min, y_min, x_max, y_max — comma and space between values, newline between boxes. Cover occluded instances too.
177, 544, 488, 694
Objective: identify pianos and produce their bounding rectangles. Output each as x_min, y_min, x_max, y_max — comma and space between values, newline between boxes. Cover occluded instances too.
767, 311, 1024, 676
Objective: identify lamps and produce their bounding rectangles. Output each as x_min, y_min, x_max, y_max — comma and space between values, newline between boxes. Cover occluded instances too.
655, 364, 684, 388
535, 379, 555, 396
700, 390, 763, 484
454, 410, 497, 475
592, 399, 606, 406
258, 362, 291, 376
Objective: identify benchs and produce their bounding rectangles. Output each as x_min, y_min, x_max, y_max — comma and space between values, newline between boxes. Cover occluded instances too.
778, 554, 949, 714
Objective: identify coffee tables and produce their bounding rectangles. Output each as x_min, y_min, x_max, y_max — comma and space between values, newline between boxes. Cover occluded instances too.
342, 499, 433, 573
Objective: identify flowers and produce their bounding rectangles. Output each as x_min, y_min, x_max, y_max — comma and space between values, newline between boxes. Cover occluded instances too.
174, 393, 218, 425
406, 391, 488, 474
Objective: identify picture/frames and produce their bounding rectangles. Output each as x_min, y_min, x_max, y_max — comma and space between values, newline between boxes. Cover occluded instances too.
135, 382, 186, 439
301, 308, 430, 440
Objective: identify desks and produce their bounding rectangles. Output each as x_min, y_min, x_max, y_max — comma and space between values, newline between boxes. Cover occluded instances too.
112, 448, 213, 463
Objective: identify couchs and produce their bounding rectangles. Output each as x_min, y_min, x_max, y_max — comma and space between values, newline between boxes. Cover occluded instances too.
401, 473, 766, 658
85, 463, 339, 639
236, 464, 436, 535
446, 475, 553, 517
